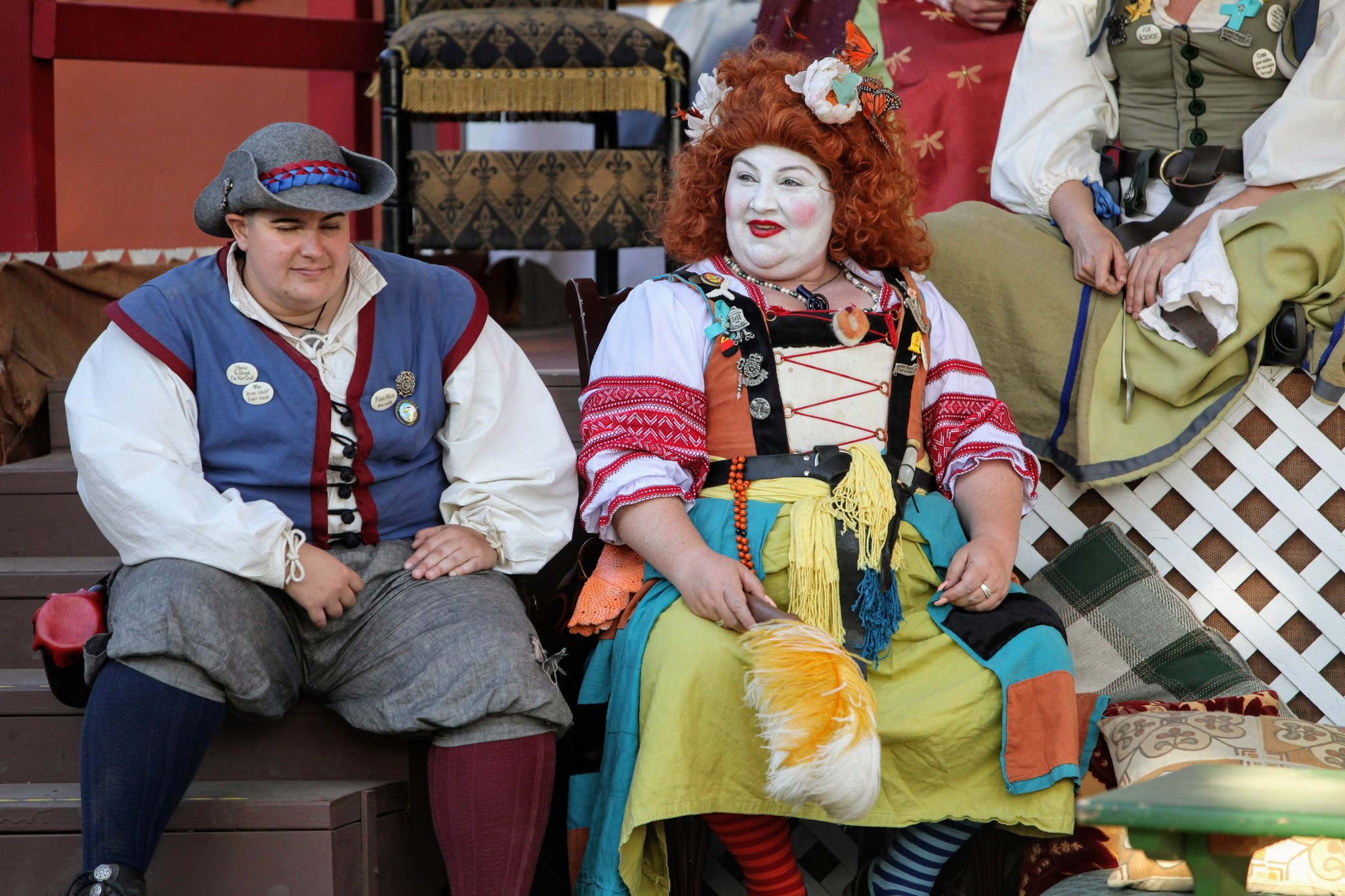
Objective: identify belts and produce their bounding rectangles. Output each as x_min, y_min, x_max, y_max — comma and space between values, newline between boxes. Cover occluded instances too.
702, 446, 939, 681
1099, 144, 1243, 252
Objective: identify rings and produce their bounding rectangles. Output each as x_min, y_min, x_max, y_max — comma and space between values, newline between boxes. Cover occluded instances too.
717, 619, 725, 628
979, 583, 992, 598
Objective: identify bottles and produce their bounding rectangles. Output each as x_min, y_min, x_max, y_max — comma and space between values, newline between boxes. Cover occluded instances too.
32, 588, 105, 707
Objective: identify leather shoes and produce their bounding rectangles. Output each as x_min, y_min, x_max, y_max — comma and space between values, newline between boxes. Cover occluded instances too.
1259, 302, 1306, 367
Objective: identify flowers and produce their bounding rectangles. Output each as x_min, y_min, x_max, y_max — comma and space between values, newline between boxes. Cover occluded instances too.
672, 66, 734, 147
785, 57, 863, 124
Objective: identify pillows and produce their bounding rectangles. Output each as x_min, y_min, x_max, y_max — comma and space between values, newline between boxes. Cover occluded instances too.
1099, 713, 1345, 896
1024, 521, 1296, 719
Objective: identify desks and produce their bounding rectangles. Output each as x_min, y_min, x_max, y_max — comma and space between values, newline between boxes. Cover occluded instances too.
1076, 761, 1345, 894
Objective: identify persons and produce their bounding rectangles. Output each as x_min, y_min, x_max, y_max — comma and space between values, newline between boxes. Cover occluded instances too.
63, 123, 580, 896
752, 0, 1034, 220
920, 0, 1345, 486
567, 20, 1082, 896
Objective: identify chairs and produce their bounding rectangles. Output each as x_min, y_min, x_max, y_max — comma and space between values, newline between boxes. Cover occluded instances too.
361, 2, 692, 295
565, 276, 1005, 896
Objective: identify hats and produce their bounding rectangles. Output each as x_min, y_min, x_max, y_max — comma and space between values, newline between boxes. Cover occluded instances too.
193, 122, 397, 238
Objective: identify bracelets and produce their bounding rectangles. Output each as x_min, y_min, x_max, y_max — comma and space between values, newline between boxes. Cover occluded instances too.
1020, 0, 1026, 26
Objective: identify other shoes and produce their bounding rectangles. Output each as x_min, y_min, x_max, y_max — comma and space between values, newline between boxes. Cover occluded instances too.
66, 863, 147, 896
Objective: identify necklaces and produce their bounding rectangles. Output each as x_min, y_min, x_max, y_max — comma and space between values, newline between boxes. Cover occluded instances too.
722, 254, 879, 314
271, 302, 327, 350
